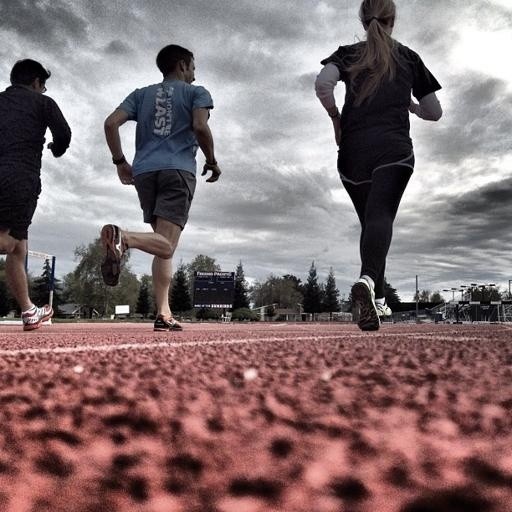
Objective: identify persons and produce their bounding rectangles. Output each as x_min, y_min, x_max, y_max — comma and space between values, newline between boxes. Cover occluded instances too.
1, 59, 73, 330
98, 44, 224, 333
315, 1, 444, 334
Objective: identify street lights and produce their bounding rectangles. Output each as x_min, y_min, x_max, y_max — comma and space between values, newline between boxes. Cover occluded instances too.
441, 282, 496, 303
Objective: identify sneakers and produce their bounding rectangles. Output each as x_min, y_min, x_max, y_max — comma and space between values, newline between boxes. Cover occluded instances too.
351, 278, 379, 331
376, 302, 392, 316
154, 314, 183, 331
22, 304, 54, 330
101, 224, 128, 286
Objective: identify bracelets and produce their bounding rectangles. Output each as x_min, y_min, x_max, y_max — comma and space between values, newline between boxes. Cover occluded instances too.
112, 155, 126, 165
206, 160, 219, 166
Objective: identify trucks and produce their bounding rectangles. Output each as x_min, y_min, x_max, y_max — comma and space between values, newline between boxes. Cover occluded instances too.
399, 311, 426, 321
